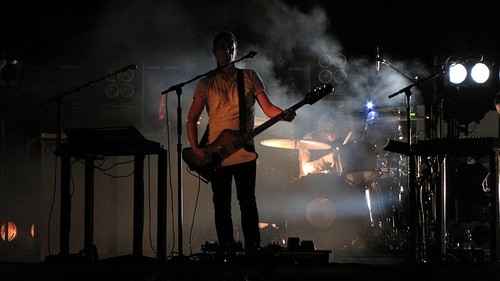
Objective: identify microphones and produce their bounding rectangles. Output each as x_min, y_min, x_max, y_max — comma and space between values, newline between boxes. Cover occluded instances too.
127, 64, 137, 69
376, 47, 380, 71
249, 51, 258, 58
465, 55, 483, 62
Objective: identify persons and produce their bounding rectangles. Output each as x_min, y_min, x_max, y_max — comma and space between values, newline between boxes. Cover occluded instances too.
297, 112, 385, 226
186, 30, 297, 255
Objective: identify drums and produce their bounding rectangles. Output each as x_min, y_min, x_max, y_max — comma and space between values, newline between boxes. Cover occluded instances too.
333, 140, 378, 184
278, 173, 371, 250
376, 151, 406, 179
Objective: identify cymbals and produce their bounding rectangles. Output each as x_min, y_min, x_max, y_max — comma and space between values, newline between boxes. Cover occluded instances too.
366, 114, 430, 122
259, 137, 332, 150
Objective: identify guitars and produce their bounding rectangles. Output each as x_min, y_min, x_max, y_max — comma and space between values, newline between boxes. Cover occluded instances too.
182, 82, 335, 183
